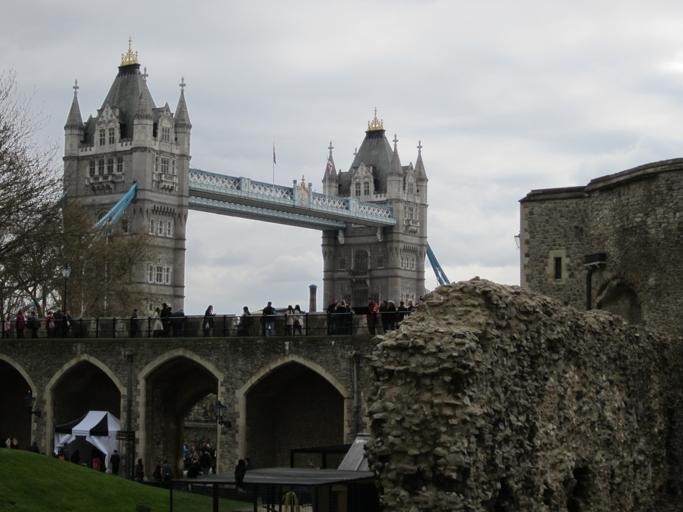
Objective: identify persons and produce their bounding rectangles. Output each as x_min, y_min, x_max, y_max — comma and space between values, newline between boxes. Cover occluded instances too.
202, 305, 215, 330
135, 457, 176, 482
366, 295, 424, 337
292, 305, 306, 336
263, 302, 275, 336
242, 307, 252, 317
6, 434, 41, 456
57, 442, 121, 475
183, 439, 217, 477
284, 306, 294, 337
4, 308, 74, 338
327, 298, 356, 335
128, 302, 187, 339
234, 458, 249, 491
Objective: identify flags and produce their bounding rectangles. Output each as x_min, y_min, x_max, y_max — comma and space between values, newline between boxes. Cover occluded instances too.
327, 160, 333, 175
273, 151, 276, 163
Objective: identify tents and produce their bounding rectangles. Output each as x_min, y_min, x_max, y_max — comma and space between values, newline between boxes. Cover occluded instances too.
54, 409, 121, 475
